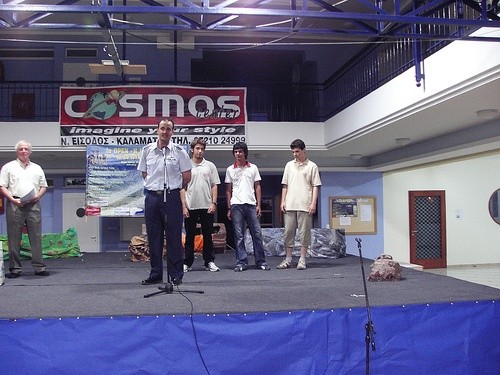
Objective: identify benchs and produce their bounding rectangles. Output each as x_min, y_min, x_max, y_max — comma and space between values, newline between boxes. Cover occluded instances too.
142, 224, 226, 255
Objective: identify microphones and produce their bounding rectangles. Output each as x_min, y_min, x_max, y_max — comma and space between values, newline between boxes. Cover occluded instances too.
161, 145, 166, 155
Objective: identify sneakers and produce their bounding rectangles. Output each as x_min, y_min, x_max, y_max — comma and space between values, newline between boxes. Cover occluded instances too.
277, 260, 294, 269
204, 261, 221, 272
296, 258, 308, 269
182, 264, 192, 274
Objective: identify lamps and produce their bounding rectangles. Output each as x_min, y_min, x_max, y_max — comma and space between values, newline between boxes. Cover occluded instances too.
101, 60, 130, 66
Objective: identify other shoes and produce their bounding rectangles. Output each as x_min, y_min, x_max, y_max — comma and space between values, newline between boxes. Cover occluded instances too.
260, 262, 270, 270
172, 277, 183, 286
234, 263, 248, 273
34, 270, 49, 276
142, 277, 163, 286
6, 273, 19, 279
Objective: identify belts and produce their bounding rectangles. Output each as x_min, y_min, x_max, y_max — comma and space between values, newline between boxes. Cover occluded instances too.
149, 188, 179, 195
11, 196, 21, 199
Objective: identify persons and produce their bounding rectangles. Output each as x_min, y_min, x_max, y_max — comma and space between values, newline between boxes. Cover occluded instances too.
0, 141, 50, 278
276, 139, 323, 270
137, 118, 193, 284
179, 140, 221, 272
224, 142, 271, 272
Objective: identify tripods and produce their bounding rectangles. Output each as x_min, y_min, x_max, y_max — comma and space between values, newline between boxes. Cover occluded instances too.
143, 155, 204, 297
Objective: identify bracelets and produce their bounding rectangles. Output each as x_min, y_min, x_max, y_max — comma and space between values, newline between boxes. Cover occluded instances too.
212, 202, 217, 205
228, 209, 231, 210
